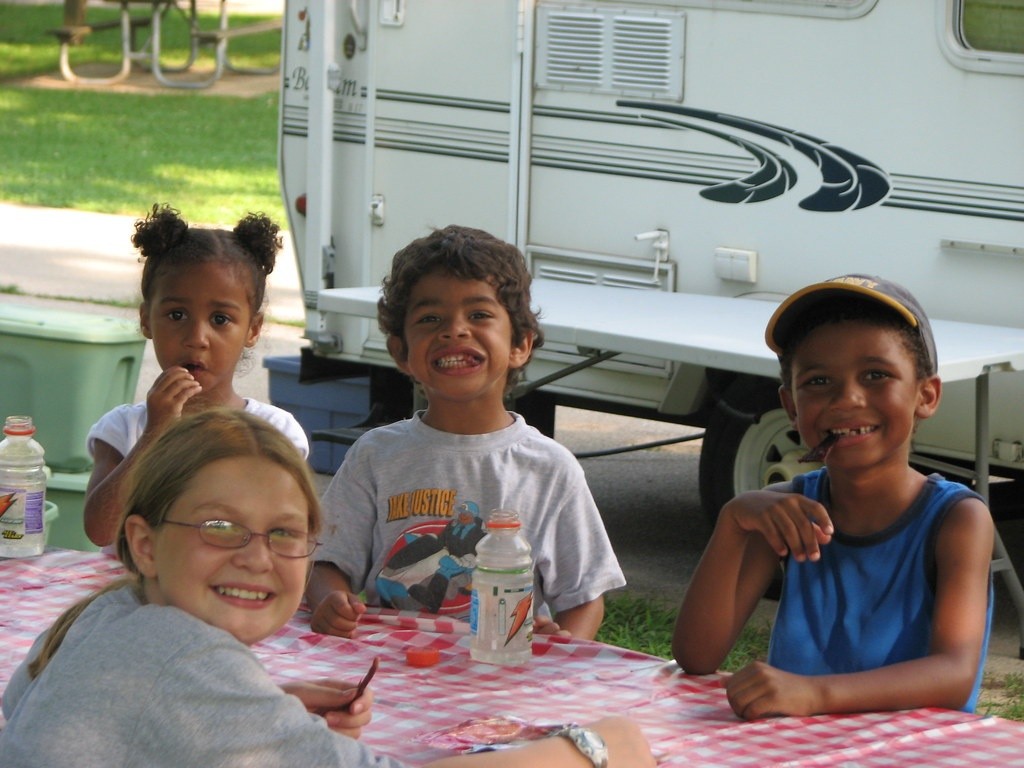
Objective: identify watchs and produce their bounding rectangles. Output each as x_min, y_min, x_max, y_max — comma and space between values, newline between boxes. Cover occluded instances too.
548, 722, 608, 768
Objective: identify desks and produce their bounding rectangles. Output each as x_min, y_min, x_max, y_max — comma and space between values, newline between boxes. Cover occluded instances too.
0, 551, 1023, 767
318, 280, 1024, 660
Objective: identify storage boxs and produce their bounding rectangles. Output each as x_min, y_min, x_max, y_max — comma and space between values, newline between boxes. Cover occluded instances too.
41, 471, 104, 556
0, 302, 148, 474
261, 353, 372, 477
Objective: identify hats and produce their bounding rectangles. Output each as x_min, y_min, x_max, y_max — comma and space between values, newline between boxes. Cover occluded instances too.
764, 272, 939, 376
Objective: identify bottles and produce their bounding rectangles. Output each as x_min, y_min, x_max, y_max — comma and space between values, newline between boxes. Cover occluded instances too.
0, 415, 47, 557
469, 509, 534, 667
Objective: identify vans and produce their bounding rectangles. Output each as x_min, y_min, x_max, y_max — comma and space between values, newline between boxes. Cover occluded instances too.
276, 0, 1022, 603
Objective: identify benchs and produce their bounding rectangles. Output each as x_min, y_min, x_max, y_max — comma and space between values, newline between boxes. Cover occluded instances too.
47, 17, 152, 86
192, 20, 283, 90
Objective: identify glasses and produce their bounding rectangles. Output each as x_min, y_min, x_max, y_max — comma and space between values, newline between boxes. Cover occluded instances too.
161, 517, 325, 559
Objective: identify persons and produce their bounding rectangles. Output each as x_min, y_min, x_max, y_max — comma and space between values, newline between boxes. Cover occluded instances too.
0, 200, 661, 768
672, 274, 995, 720
